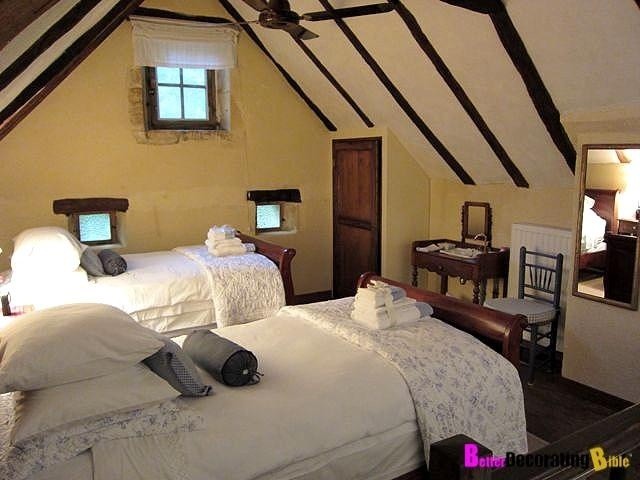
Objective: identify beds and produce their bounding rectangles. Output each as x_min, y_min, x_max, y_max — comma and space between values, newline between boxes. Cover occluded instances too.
1, 230, 528, 480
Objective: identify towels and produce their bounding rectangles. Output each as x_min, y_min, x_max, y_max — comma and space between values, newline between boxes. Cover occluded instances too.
207, 224, 236, 240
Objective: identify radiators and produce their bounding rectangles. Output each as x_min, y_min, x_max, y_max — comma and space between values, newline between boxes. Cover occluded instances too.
506, 222, 572, 353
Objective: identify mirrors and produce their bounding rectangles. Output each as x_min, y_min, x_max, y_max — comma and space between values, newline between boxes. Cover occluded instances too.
461, 201, 493, 241
571, 142, 640, 313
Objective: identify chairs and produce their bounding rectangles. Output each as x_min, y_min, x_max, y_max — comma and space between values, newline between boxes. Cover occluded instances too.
483, 246, 564, 384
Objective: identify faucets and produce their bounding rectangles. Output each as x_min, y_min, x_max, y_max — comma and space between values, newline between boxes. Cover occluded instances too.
475, 233, 488, 253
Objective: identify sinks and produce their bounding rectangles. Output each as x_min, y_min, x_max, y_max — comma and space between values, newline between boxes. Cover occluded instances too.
440, 247, 496, 259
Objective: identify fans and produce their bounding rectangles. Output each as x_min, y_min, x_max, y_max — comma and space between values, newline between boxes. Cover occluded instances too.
211, 0, 393, 41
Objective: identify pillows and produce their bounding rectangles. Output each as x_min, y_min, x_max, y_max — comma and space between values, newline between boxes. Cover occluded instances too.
9, 226, 89, 278
